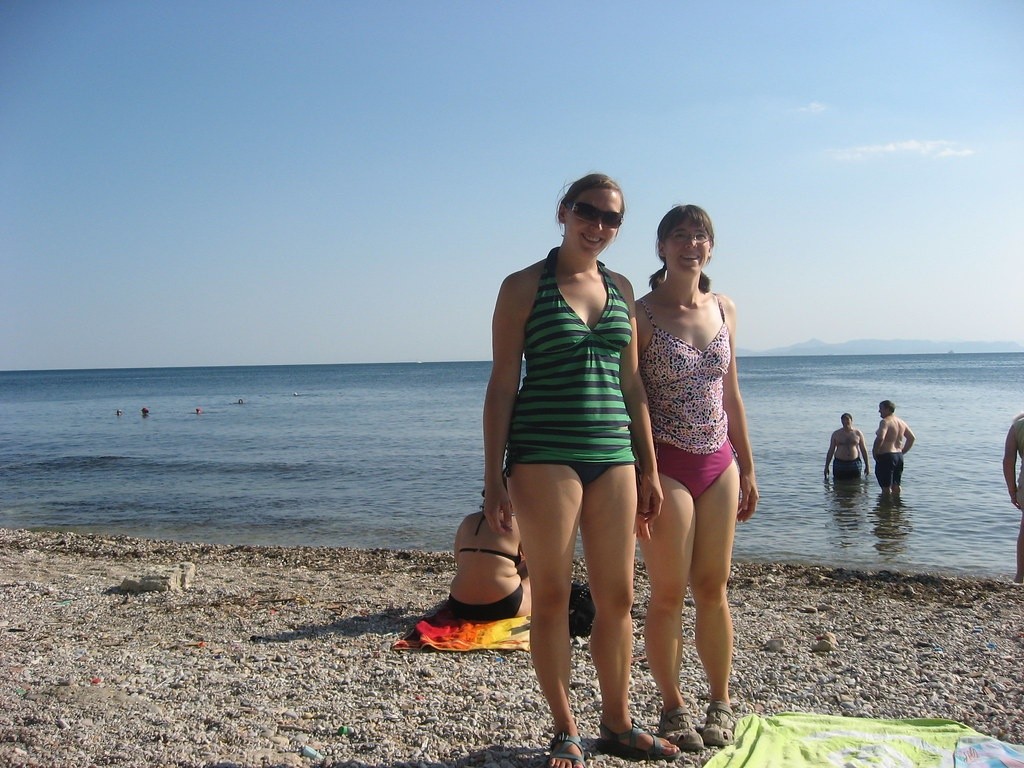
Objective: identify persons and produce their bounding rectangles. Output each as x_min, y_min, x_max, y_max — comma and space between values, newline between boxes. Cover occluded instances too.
447, 474, 532, 620
1003, 413, 1024, 583
117, 409, 122, 415
634, 204, 759, 751
824, 413, 869, 478
196, 408, 202, 414
872, 400, 915, 488
483, 175, 681, 768
142, 407, 148, 414
239, 399, 243, 403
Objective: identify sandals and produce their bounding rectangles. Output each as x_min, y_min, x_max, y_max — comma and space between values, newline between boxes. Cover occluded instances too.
547, 733, 586, 767
660, 706, 705, 752
702, 698, 735, 745
599, 717, 681, 761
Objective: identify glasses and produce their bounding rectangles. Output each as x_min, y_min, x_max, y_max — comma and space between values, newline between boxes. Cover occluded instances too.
664, 230, 713, 245
561, 200, 624, 228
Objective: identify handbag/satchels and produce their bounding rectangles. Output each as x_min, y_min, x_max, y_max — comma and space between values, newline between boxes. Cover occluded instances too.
568, 579, 596, 637
